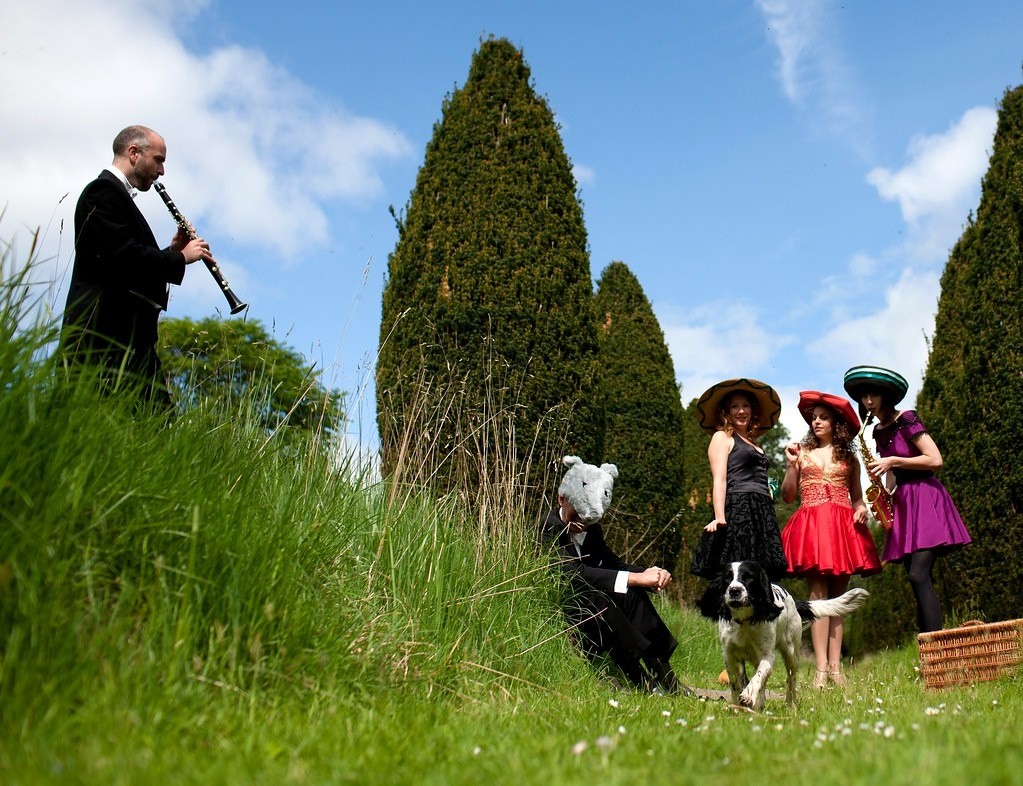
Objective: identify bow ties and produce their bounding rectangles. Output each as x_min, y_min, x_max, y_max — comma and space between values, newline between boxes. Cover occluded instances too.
571, 531, 587, 545
129, 188, 137, 198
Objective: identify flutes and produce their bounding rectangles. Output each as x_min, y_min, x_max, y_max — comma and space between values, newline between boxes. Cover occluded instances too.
152, 178, 248, 316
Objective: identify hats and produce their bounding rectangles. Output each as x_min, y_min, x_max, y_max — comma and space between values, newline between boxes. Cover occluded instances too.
844, 365, 908, 405
696, 378, 781, 439
797, 390, 860, 442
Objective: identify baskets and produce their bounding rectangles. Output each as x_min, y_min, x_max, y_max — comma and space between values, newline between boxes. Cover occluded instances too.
916, 619, 1023, 693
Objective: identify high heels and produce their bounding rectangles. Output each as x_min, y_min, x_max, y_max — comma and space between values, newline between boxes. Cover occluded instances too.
830, 670, 844, 686
814, 665, 828, 691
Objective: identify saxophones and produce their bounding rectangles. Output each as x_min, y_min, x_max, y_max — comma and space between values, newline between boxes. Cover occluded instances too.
856, 407, 896, 532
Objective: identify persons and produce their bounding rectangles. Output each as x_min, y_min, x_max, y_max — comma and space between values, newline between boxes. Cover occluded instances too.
844, 365, 972, 633
57, 125, 217, 428
781, 391, 881, 693
690, 379, 788, 682
536, 456, 697, 699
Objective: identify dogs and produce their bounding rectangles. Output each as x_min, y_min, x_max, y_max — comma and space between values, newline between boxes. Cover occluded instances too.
700, 560, 872, 709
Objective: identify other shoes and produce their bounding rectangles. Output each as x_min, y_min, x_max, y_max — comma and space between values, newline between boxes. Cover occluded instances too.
652, 685, 668, 696
669, 681, 697, 701
